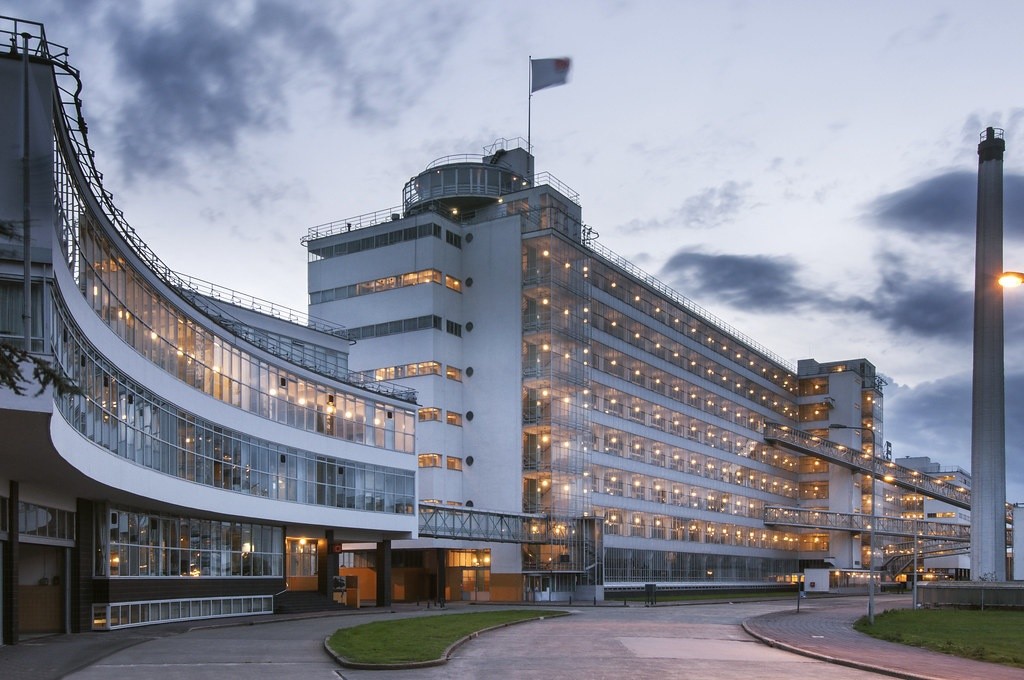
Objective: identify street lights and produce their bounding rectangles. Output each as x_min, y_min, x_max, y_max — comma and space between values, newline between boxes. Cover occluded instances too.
884, 475, 919, 611
828, 422, 876, 621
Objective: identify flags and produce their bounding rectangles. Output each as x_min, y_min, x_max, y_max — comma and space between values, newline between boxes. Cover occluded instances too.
532, 58, 570, 93
